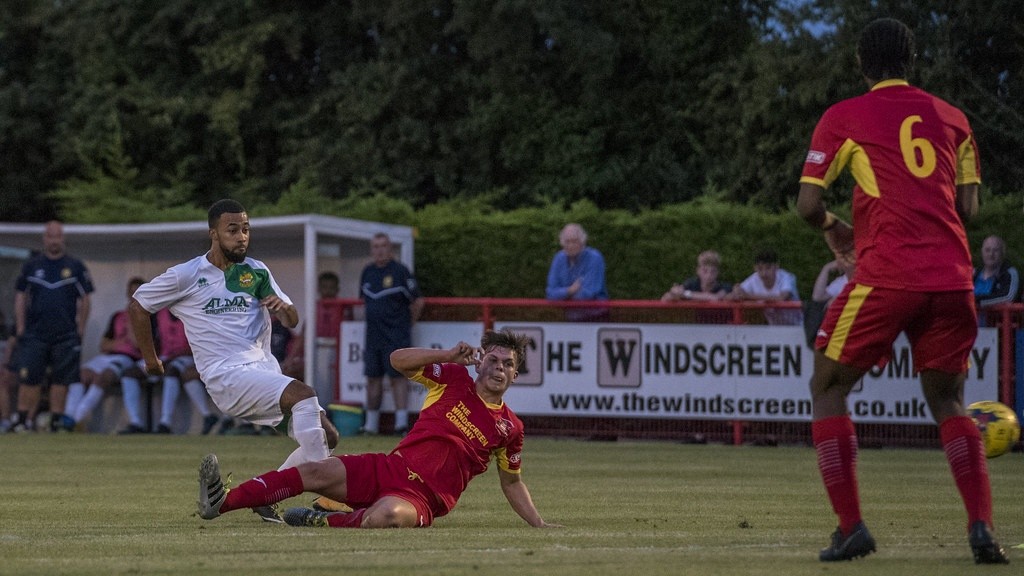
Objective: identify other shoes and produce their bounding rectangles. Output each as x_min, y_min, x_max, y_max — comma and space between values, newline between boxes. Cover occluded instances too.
201, 415, 217, 434
120, 425, 142, 434
156, 425, 170, 432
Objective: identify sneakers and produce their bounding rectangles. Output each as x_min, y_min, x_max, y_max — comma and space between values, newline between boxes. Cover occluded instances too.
251, 504, 282, 522
284, 506, 340, 527
819, 521, 877, 561
310, 495, 353, 513
196, 453, 232, 519
968, 521, 1010, 564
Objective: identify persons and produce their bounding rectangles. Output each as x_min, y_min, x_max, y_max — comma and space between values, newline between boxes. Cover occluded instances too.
129, 200, 353, 524
121, 309, 216, 434
723, 253, 804, 444
0, 222, 95, 433
55, 277, 147, 432
359, 233, 425, 437
283, 270, 351, 375
811, 260, 848, 310
798, 19, 1011, 563
221, 309, 290, 435
972, 235, 1020, 327
662, 251, 733, 441
546, 224, 618, 440
198, 330, 563, 528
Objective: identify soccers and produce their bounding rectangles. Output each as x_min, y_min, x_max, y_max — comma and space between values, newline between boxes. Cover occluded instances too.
965, 400, 1021, 459
